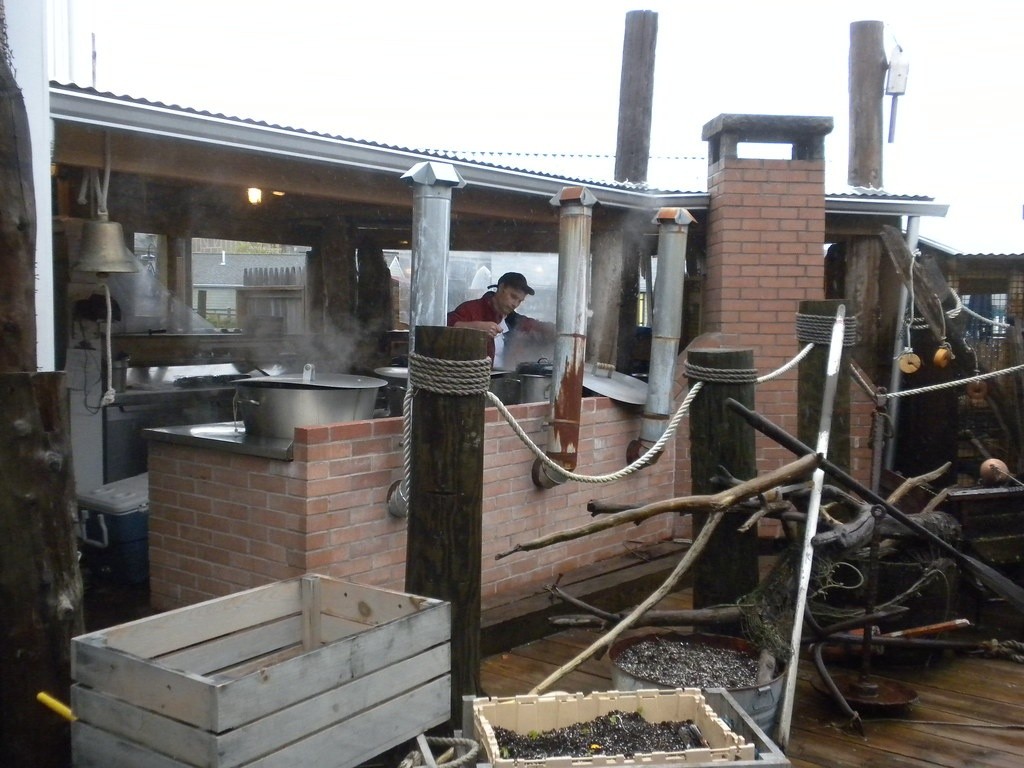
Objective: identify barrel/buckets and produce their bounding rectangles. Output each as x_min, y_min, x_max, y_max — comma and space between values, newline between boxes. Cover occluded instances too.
607, 630, 789, 735
102, 360, 129, 392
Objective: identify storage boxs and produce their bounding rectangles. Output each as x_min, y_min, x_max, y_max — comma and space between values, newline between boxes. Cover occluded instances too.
72, 572, 452, 768
76, 471, 150, 591
457, 687, 792, 768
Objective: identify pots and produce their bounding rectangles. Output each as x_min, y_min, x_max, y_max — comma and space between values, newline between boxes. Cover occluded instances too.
506, 357, 554, 403
230, 364, 389, 438
373, 366, 512, 414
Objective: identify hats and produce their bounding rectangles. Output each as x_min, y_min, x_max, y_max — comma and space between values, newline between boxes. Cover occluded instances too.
487, 272, 535, 296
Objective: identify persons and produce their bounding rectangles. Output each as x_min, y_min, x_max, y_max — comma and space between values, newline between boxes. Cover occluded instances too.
447, 272, 557, 368
